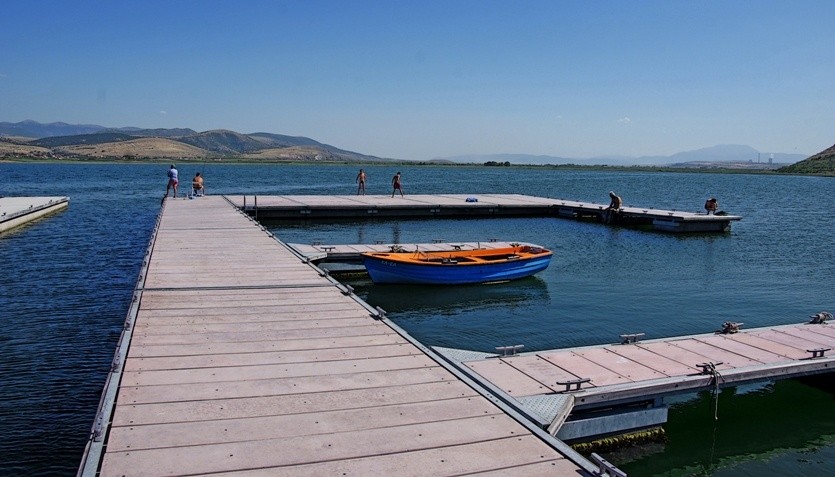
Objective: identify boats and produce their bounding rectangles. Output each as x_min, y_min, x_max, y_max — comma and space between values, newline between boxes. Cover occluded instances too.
358, 244, 554, 284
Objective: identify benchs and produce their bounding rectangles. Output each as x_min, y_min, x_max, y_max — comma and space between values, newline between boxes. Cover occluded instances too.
515, 252, 533, 256
464, 257, 485, 262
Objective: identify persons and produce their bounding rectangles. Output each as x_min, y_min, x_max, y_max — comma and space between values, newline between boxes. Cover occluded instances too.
392, 172, 404, 198
193, 173, 205, 195
165, 164, 179, 198
705, 199, 718, 215
356, 169, 367, 195
605, 191, 622, 224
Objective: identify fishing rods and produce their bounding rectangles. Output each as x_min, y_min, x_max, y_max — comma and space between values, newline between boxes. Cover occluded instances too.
201, 150, 209, 177
